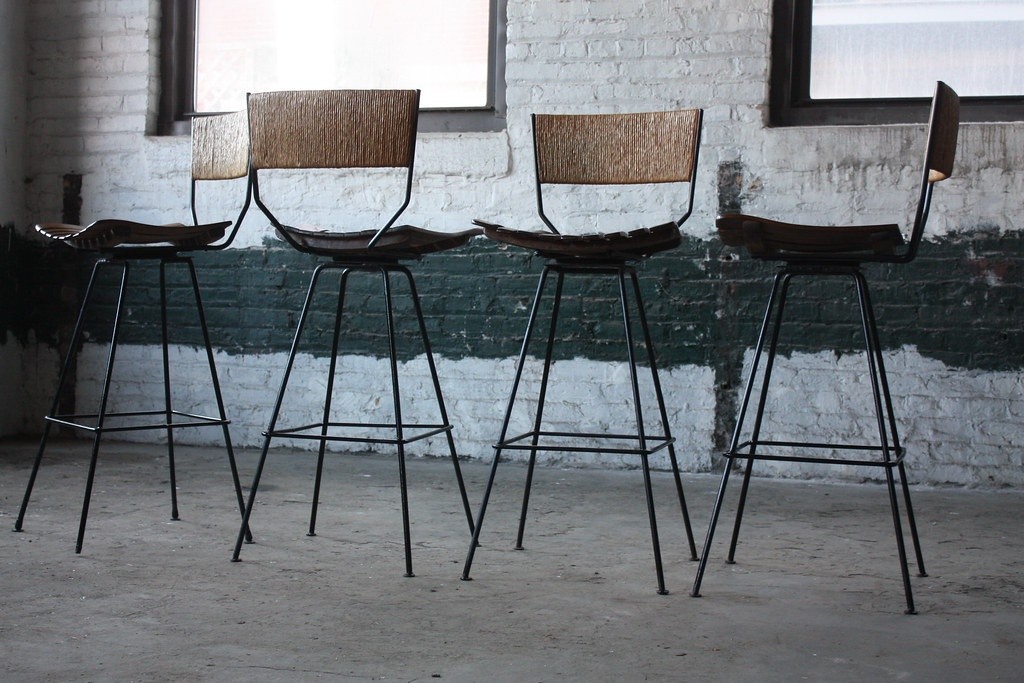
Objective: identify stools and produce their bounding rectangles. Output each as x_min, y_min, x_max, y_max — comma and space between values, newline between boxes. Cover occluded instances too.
690, 81, 963, 615
11, 110, 256, 555
228, 88, 483, 579
458, 108, 703, 596
